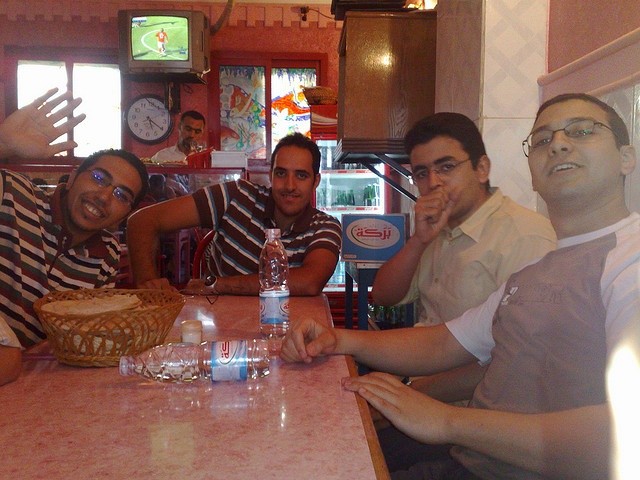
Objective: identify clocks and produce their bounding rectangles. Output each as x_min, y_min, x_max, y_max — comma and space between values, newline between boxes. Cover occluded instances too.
124, 95, 175, 145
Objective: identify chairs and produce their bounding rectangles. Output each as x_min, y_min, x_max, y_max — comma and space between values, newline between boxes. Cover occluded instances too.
115, 243, 134, 284
191, 231, 214, 281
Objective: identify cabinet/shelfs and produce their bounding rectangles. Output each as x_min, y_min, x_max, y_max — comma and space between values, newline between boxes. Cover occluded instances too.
122, 72, 207, 114
331, 11, 434, 202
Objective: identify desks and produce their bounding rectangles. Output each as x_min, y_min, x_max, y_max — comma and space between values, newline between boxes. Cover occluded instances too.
344, 257, 414, 376
159, 228, 193, 284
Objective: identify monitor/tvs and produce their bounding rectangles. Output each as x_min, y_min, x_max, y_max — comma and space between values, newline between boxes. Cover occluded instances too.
118, 9, 210, 77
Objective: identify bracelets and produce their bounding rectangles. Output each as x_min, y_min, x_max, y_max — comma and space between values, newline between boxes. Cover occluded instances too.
401, 378, 411, 386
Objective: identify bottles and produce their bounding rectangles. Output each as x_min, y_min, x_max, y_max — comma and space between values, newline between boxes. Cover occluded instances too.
119, 339, 270, 385
327, 258, 346, 283
179, 319, 203, 344
367, 302, 404, 329
317, 188, 355, 206
258, 227, 290, 339
362, 184, 379, 207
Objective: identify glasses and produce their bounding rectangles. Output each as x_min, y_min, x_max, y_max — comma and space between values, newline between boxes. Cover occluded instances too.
86, 170, 135, 204
522, 119, 619, 158
412, 160, 471, 182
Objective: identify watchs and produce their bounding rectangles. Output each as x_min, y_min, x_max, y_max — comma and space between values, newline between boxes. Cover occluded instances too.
204, 276, 220, 294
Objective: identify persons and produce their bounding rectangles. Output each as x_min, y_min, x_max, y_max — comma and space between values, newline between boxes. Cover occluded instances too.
280, 93, 640, 479
0, 87, 148, 353
1, 316, 27, 387
370, 111, 557, 473
151, 110, 206, 165
125, 131, 343, 306
155, 27, 169, 56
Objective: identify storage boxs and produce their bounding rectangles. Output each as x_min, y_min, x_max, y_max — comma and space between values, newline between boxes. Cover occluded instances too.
341, 212, 410, 263
